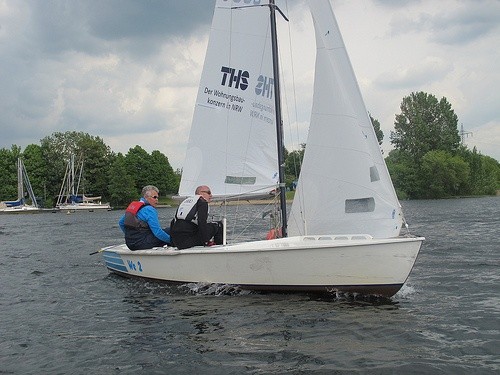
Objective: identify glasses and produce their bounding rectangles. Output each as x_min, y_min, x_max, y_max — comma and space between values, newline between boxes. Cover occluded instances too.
198, 190, 212, 194
147, 194, 160, 200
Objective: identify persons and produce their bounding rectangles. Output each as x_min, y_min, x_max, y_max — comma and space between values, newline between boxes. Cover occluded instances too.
170, 185, 228, 250
119, 185, 171, 250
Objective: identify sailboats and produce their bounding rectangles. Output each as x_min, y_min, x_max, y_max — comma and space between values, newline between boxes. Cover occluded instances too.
102, 0, 425, 299
0, 152, 111, 212
